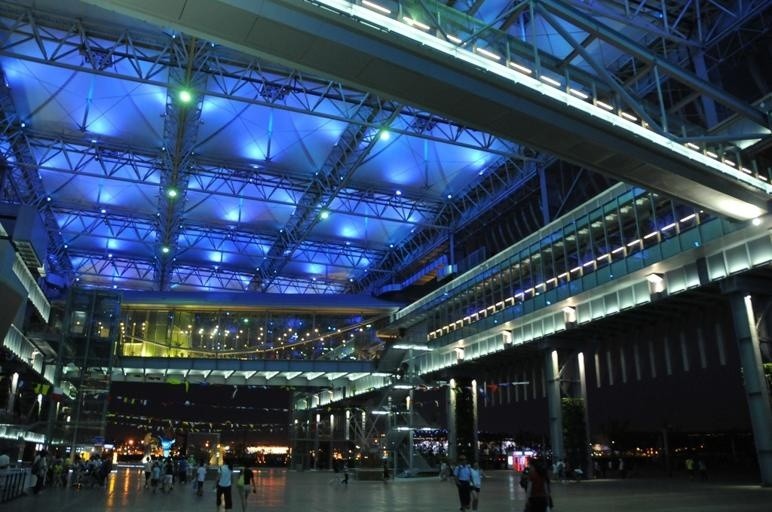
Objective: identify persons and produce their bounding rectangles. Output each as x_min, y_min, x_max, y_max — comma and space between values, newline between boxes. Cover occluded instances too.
28, 447, 114, 495
516, 454, 624, 512
686, 455, 698, 480
699, 459, 709, 480
384, 460, 390, 483
438, 453, 492, 511
0, 450, 10, 470
341, 461, 349, 485
142, 452, 256, 512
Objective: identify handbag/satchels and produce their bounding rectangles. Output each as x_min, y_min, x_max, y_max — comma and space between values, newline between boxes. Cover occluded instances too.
236, 474, 244, 487
